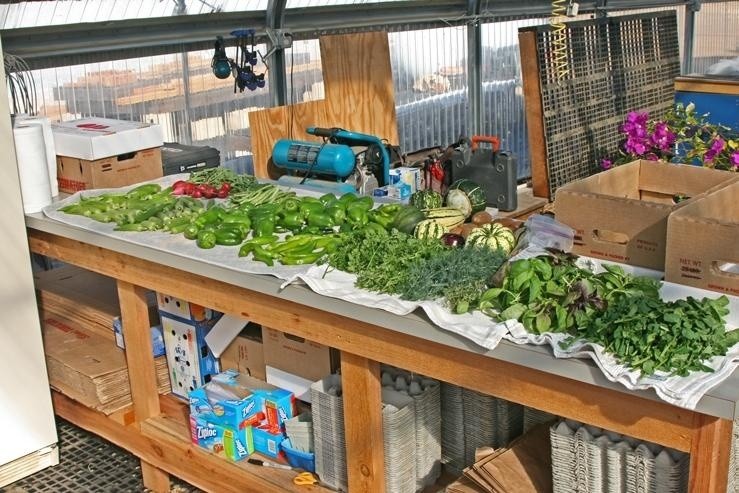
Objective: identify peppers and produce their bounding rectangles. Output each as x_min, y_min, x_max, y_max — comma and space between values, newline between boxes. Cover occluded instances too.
184, 179, 401, 266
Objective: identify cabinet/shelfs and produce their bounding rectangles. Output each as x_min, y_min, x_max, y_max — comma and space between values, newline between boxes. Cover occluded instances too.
22, 184, 737, 491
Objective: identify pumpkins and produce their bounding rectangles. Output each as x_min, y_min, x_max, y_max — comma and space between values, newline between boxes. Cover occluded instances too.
393, 178, 517, 258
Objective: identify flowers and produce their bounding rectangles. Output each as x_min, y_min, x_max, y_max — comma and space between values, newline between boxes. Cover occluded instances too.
590, 97, 738, 172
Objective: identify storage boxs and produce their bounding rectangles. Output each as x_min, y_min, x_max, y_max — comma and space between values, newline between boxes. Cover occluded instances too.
43, 113, 163, 196
188, 325, 333, 462
552, 154, 738, 269
663, 178, 739, 294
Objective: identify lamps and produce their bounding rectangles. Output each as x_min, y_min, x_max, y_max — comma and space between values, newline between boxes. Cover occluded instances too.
209, 24, 298, 93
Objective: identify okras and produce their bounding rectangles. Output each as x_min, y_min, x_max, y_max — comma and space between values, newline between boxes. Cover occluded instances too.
56, 184, 204, 234
